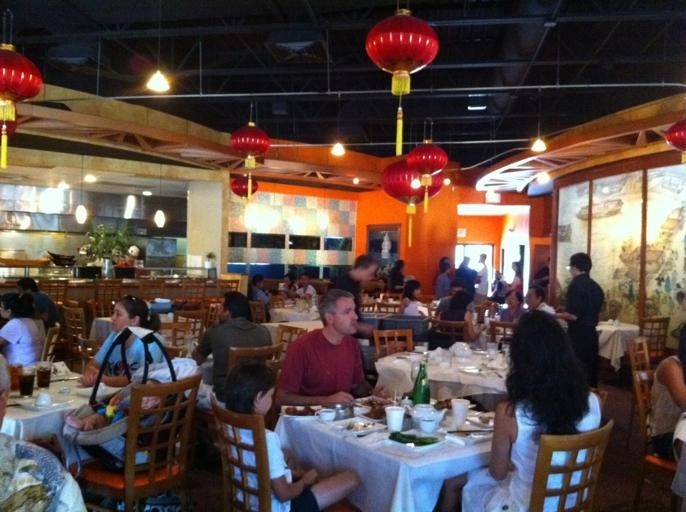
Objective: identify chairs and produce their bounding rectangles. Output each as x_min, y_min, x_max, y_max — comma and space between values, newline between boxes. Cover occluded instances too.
0, 261, 686, 512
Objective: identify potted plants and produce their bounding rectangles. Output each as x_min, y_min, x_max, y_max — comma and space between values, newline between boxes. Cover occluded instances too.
81, 220, 138, 279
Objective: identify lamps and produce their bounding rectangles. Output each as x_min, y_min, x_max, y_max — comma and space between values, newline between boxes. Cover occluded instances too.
144, 1, 171, 96
330, 93, 346, 155
530, 89, 547, 152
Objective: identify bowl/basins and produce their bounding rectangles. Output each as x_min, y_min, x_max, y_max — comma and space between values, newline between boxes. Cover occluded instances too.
46, 251, 77, 266
148, 300, 172, 313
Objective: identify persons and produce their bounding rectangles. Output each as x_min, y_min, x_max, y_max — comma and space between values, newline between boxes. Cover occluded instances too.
649, 324, 686, 459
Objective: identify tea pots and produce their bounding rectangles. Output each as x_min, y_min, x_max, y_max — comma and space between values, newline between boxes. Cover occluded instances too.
404, 401, 448, 429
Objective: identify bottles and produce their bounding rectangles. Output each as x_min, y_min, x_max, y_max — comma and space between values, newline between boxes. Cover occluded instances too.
410, 358, 432, 406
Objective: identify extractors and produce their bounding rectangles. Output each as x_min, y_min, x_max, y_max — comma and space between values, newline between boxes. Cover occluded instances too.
0, 183, 187, 239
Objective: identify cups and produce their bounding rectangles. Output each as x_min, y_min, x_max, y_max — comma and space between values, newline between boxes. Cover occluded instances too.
413, 340, 511, 375
34, 392, 50, 406
8, 362, 52, 399
385, 404, 404, 435
451, 397, 471, 428
314, 408, 336, 423
419, 417, 436, 434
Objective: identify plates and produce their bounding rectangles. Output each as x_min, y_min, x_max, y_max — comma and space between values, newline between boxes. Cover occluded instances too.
30, 402, 54, 412
75, 386, 122, 398
353, 394, 391, 422
331, 415, 494, 449
283, 404, 317, 420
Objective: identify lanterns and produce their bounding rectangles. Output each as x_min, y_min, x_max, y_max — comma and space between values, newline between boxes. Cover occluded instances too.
364, 9, 441, 156
406, 138, 449, 213
380, 159, 445, 249
664, 119, 686, 165
1, 42, 43, 170
229, 121, 273, 198
231, 176, 259, 210
